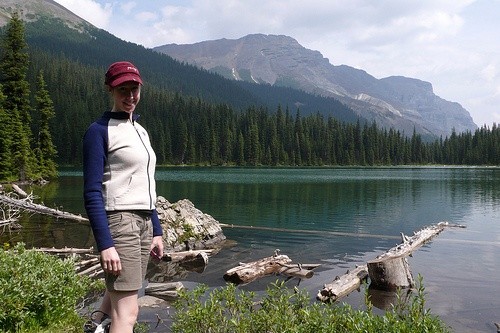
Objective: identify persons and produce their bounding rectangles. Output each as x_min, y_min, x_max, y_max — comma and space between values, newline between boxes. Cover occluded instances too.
82, 61, 164, 333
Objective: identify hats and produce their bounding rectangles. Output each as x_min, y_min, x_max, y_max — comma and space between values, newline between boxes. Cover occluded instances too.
105, 61, 143, 86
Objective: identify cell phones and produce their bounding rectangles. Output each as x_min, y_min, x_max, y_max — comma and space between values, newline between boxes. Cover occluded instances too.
150, 249, 162, 264
